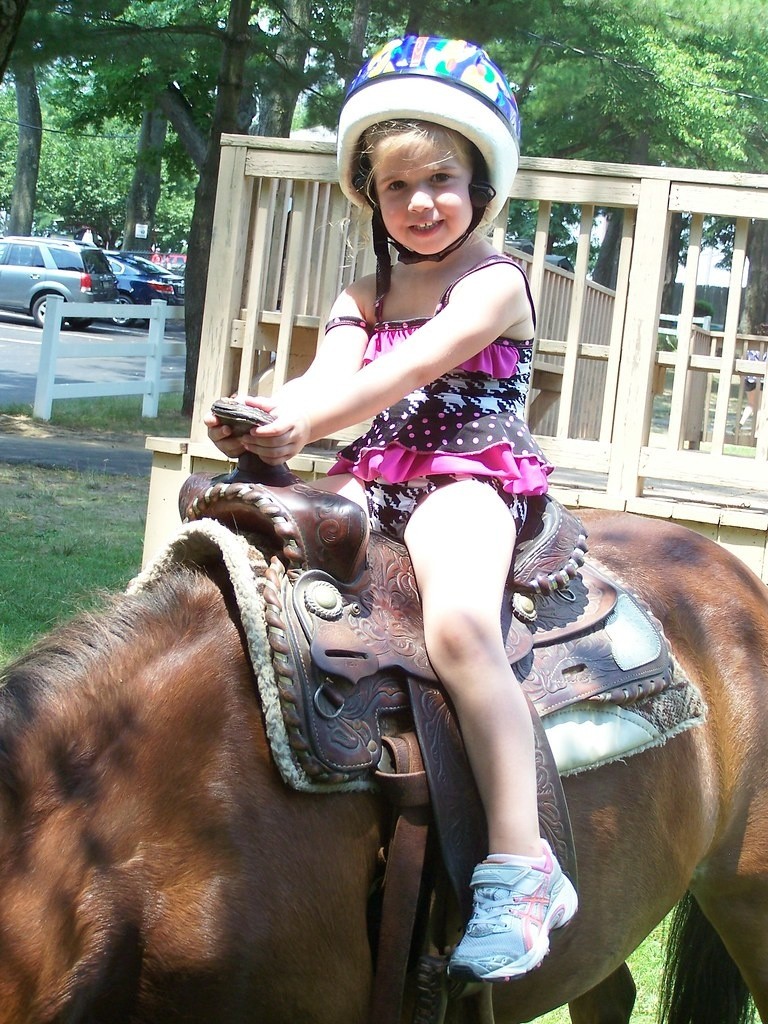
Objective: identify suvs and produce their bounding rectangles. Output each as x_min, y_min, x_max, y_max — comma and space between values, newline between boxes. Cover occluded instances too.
0, 234, 120, 330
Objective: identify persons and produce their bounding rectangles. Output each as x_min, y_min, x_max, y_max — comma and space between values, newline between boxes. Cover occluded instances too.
203, 37, 580, 982
730, 323, 768, 437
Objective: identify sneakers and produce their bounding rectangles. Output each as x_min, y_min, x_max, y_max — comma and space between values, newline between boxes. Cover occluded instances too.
447, 838, 578, 983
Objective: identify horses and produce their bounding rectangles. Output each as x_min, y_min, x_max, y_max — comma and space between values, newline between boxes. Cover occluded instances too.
1, 498, 767, 1024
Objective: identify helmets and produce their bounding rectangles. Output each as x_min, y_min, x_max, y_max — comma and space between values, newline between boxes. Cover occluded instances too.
337, 35, 521, 227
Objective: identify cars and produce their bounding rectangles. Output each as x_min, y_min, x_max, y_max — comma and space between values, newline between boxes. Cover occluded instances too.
152, 252, 187, 277
100, 247, 186, 326
